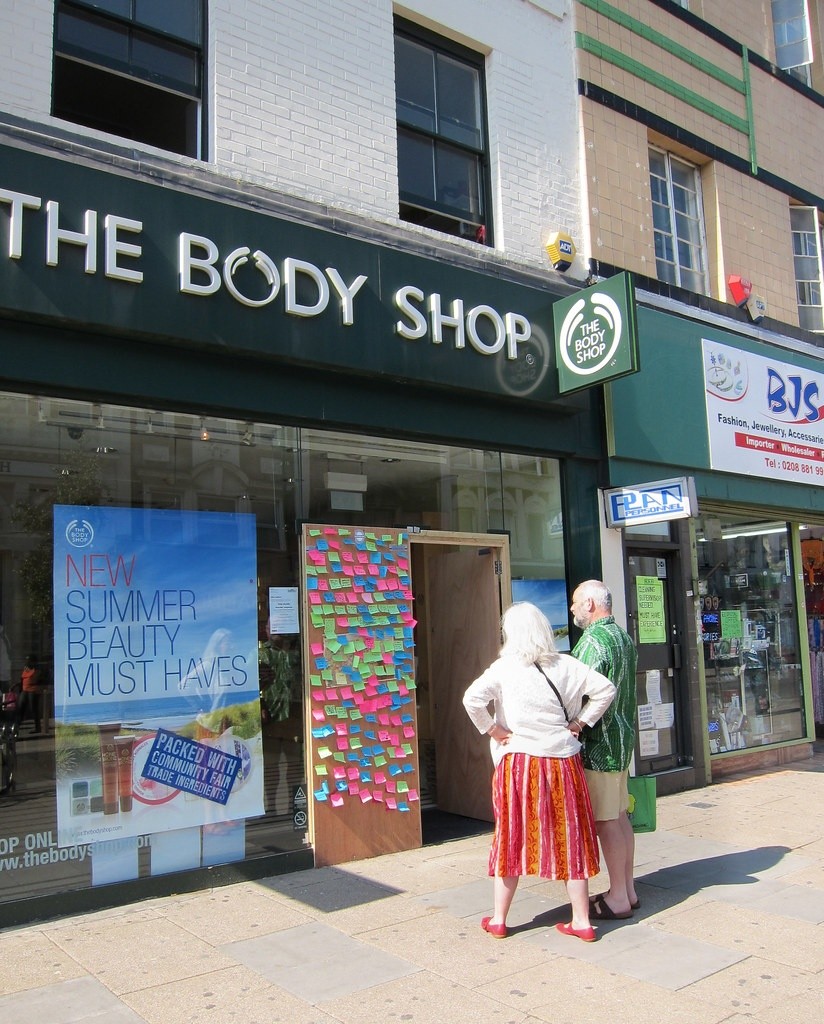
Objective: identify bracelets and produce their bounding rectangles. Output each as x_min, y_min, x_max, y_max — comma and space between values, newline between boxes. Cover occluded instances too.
573, 720, 582, 731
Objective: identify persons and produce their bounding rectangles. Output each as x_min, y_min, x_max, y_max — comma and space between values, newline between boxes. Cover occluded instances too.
13, 654, 44, 734
570, 580, 640, 919
177, 628, 236, 738
463, 600, 617, 942
247, 619, 304, 813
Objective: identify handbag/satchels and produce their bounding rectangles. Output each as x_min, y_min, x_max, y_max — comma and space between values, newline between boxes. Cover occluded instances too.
625, 769, 657, 832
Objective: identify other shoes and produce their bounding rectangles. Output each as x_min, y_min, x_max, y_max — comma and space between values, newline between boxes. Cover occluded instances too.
556, 922, 596, 942
482, 916, 507, 939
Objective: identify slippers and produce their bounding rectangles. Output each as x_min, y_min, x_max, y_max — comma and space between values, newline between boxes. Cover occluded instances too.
589, 890, 641, 910
589, 899, 633, 920
